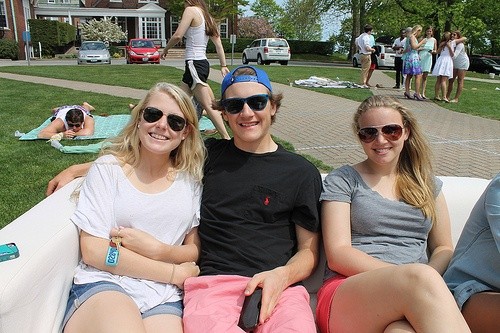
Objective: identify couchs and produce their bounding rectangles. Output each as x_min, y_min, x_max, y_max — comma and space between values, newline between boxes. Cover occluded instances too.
0, 175, 492, 333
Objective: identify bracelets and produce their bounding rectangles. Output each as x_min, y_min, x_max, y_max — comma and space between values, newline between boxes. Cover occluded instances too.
168, 264, 175, 285
221, 66, 227, 67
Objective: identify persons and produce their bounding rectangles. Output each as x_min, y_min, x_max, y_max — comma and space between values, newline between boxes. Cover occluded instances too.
61, 84, 204, 333
160, 0, 231, 142
316, 95, 473, 333
38, 101, 95, 138
47, 65, 323, 333
442, 173, 500, 333
392, 25, 470, 103
355, 24, 377, 90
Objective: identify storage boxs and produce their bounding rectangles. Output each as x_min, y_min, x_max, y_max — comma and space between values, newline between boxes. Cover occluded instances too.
0, 243, 20, 263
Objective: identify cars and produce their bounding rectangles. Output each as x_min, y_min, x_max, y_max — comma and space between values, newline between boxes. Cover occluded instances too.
76, 41, 111, 64
125, 38, 161, 64
352, 45, 396, 69
468, 56, 500, 75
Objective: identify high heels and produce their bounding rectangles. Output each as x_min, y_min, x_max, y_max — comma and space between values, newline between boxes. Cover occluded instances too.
413, 93, 423, 100
404, 92, 414, 99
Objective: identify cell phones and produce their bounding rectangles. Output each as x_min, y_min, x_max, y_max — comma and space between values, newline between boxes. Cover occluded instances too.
238, 287, 262, 330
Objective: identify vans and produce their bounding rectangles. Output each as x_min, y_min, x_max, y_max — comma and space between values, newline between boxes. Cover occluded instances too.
242, 38, 292, 65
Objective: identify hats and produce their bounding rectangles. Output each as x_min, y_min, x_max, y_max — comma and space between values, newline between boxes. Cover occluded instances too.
221, 65, 272, 95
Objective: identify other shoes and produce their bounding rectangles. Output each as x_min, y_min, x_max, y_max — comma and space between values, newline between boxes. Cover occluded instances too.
393, 86, 400, 89
449, 99, 458, 103
434, 98, 442, 101
422, 96, 426, 99
362, 85, 369, 88
441, 99, 449, 103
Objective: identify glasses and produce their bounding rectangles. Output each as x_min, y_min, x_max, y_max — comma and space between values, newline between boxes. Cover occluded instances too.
221, 94, 272, 114
68, 123, 81, 127
454, 34, 456, 36
357, 124, 405, 143
140, 107, 187, 132
